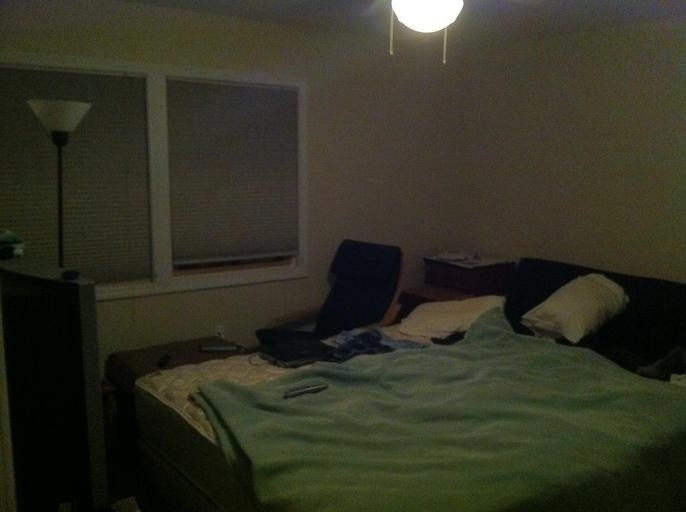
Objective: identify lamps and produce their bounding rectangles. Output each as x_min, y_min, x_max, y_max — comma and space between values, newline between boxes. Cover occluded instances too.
30, 99, 92, 269
374, 1, 471, 66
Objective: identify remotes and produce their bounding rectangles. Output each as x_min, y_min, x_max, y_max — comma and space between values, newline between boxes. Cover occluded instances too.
284, 383, 328, 398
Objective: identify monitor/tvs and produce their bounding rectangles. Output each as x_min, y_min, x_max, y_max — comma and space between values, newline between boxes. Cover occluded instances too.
0, 256, 108, 493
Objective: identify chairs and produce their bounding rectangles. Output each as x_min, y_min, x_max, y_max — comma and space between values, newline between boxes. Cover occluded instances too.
251, 237, 403, 357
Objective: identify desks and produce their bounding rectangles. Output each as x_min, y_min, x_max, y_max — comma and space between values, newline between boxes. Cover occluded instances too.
108, 336, 247, 503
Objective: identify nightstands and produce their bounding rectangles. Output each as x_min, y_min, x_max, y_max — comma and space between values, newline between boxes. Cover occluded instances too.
399, 251, 515, 313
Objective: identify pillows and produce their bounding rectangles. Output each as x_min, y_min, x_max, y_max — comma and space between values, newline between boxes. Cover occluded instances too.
522, 272, 634, 345
615, 282, 685, 360
503, 261, 577, 318
400, 293, 506, 341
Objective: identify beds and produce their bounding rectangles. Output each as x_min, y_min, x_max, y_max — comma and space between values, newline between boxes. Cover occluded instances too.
124, 257, 684, 512
